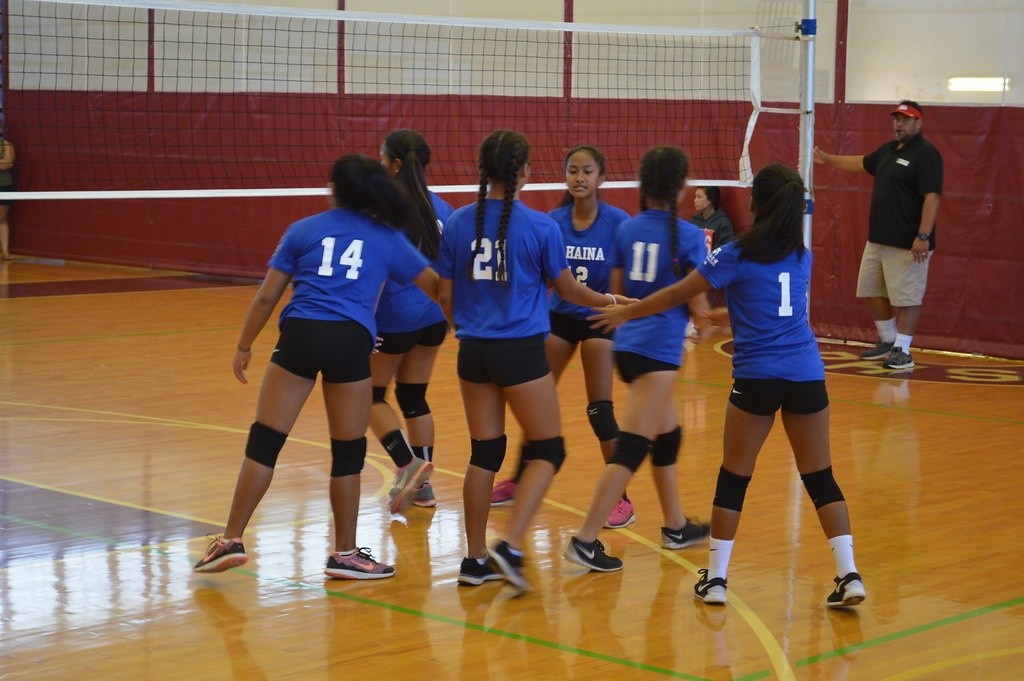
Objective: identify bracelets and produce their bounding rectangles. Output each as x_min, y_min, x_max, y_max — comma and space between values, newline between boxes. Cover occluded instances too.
237, 343, 250, 352
605, 293, 617, 304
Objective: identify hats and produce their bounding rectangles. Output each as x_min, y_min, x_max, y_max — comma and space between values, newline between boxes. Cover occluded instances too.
890, 103, 921, 121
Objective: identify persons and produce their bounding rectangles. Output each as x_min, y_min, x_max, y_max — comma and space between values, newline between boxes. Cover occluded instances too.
367, 130, 456, 514
0, 137, 19, 260
586, 162, 866, 608
440, 130, 641, 590
490, 143, 637, 529
562, 145, 712, 571
194, 154, 439, 579
813, 99, 944, 369
684, 187, 734, 338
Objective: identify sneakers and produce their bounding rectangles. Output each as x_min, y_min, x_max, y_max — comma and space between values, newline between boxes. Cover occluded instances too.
563, 537, 622, 573
457, 578, 507, 610
411, 485, 436, 508
825, 569, 866, 606
324, 575, 396, 608
660, 516, 712, 550
826, 603, 864, 662
490, 479, 518, 508
324, 551, 395, 580
390, 456, 433, 514
693, 597, 726, 630
693, 567, 727, 604
488, 540, 529, 591
603, 498, 636, 528
193, 532, 247, 573
859, 338, 897, 360
883, 346, 915, 371
563, 565, 621, 606
456, 556, 502, 585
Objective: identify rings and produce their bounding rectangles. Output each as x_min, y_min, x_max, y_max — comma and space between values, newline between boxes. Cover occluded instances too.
922, 253, 925, 255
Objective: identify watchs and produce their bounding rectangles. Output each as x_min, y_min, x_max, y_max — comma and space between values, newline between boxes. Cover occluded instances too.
917, 233, 929, 240
